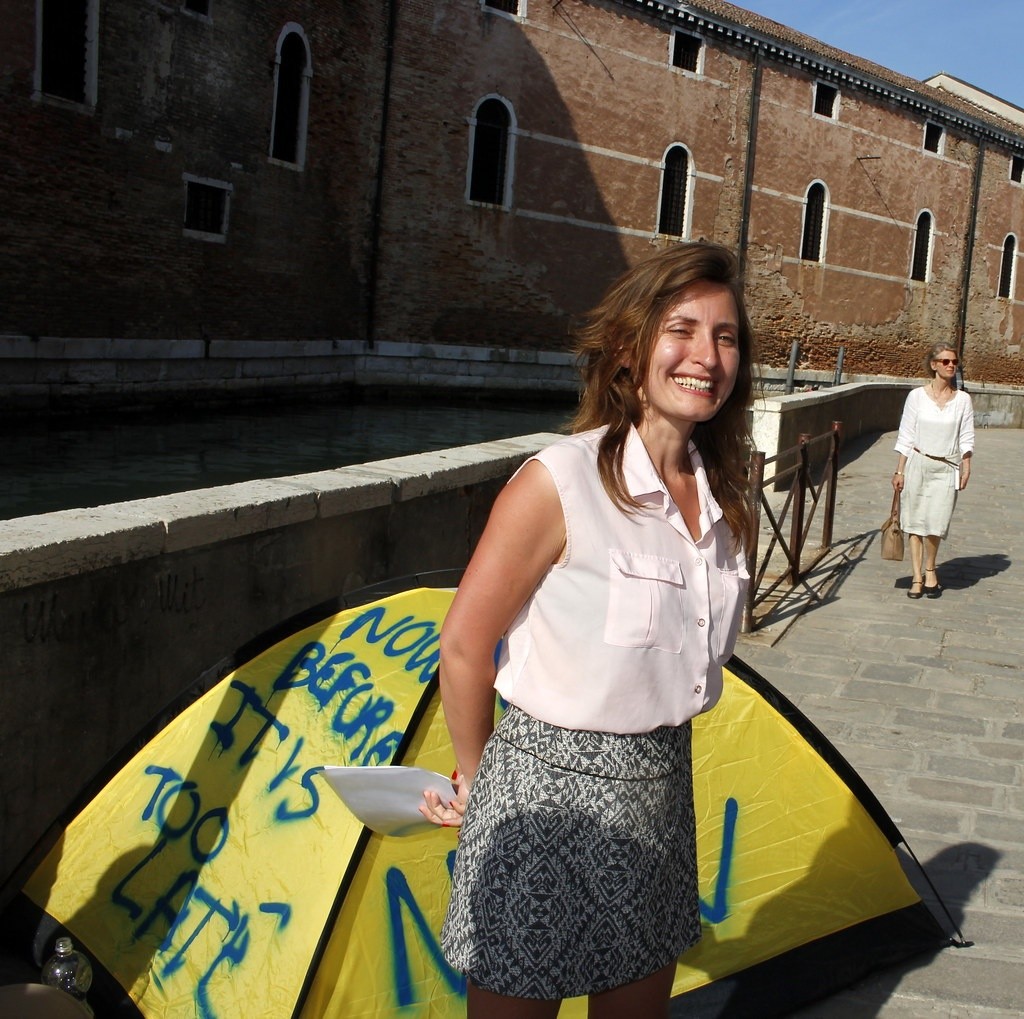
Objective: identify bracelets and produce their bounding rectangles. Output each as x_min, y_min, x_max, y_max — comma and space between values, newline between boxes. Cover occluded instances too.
895, 472, 904, 476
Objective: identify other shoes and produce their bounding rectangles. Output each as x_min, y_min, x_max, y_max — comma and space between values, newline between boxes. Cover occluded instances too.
924, 569, 942, 598
907, 581, 923, 598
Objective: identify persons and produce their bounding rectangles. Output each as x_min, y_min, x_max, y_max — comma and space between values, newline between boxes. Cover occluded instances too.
417, 241, 758, 1019
891, 341, 974, 600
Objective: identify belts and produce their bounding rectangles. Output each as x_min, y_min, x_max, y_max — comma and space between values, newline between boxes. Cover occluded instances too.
914, 447, 958, 466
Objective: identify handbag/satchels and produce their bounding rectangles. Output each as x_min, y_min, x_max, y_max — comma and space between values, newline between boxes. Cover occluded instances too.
881, 490, 904, 561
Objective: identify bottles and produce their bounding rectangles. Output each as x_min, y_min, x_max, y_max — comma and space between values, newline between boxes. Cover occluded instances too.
40, 937, 94, 1017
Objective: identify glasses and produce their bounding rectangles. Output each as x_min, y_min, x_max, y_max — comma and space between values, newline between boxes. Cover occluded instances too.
933, 358, 959, 366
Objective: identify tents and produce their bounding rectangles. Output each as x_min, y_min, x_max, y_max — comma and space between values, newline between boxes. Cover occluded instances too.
1, 562, 976, 1019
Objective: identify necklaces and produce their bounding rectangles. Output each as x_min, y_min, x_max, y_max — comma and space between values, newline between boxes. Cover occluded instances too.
931, 380, 952, 404
